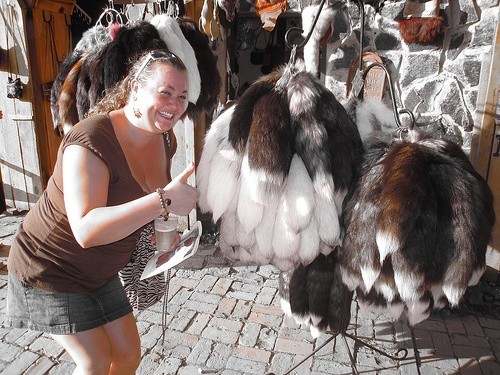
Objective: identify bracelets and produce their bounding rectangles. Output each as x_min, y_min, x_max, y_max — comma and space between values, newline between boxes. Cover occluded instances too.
157, 188, 171, 222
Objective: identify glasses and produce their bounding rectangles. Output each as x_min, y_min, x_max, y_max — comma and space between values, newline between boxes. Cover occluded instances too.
134, 51, 183, 80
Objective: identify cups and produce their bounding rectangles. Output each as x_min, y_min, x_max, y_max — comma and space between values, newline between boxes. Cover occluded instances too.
155, 218, 177, 251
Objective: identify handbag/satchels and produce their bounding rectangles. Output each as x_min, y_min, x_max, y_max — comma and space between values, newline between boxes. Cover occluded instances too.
7, 77, 24, 98
117, 221, 167, 311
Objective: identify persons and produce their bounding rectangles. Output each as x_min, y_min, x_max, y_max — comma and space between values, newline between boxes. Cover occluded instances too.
3, 49, 200, 375
